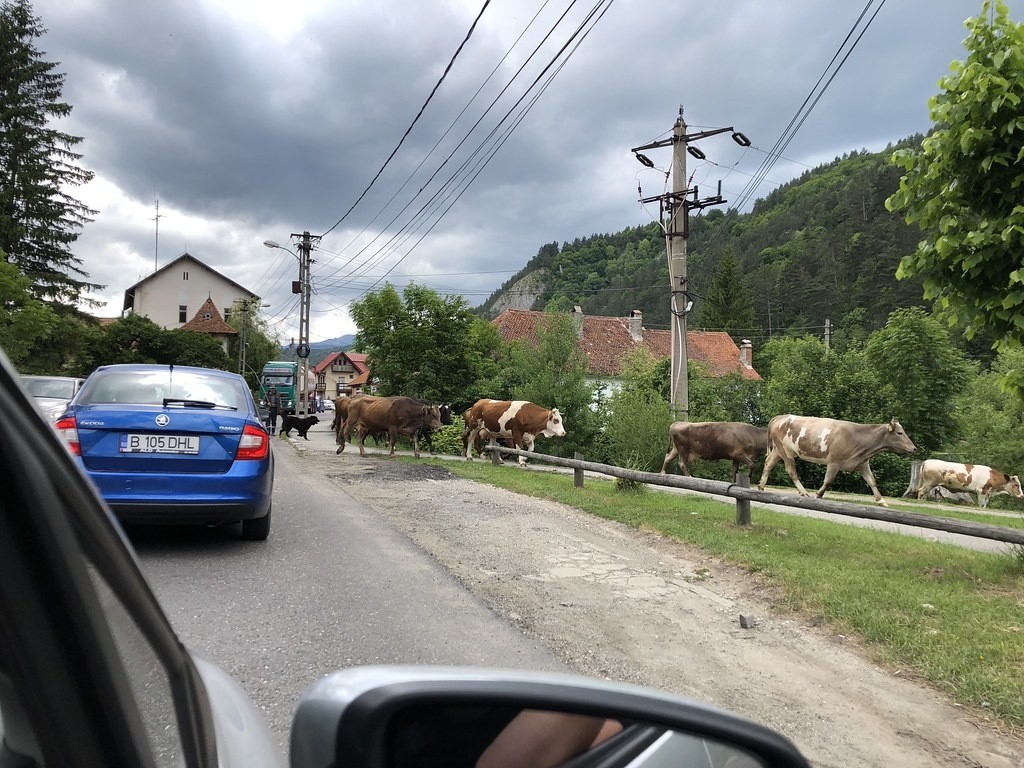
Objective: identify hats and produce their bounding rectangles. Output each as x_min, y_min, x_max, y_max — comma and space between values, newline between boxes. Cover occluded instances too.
270, 384, 276, 389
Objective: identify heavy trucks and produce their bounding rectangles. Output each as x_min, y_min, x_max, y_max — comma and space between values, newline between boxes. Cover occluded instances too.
259, 360, 318, 416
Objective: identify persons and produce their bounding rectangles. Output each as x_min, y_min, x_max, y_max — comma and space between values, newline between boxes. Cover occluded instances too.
263, 384, 284, 436
476, 711, 643, 767
318, 396, 325, 413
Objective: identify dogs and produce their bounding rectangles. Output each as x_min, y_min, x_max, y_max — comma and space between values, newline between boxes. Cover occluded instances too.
279, 412, 320, 441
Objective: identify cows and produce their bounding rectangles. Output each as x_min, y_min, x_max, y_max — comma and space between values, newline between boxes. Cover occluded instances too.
757, 413, 918, 508
463, 399, 567, 467
331, 393, 444, 460
662, 421, 768, 483
918, 459, 1024, 508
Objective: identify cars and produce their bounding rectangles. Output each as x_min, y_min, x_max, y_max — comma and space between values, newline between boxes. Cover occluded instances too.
54, 364, 275, 539
19, 375, 86, 429
323, 399, 336, 411
315, 395, 326, 412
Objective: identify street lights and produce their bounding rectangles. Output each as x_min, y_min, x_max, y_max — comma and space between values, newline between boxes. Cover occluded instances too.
238, 304, 272, 376
263, 238, 313, 418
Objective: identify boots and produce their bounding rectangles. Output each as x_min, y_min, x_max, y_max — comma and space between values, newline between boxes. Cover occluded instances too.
272, 428, 276, 436
267, 427, 270, 435
